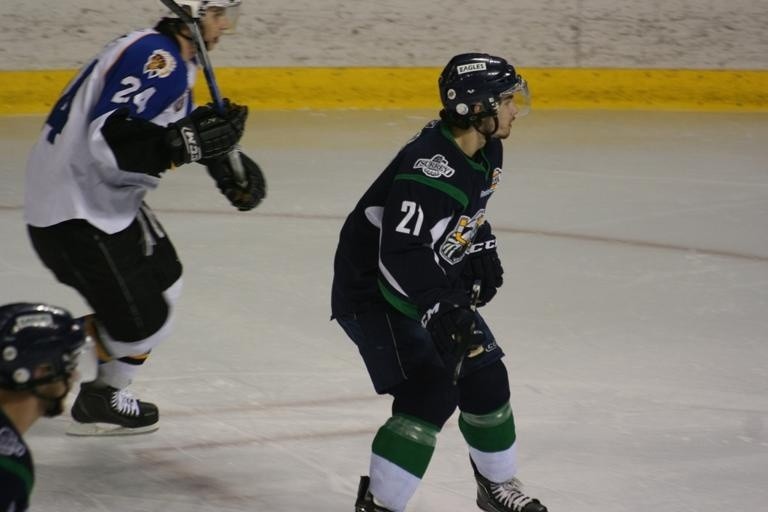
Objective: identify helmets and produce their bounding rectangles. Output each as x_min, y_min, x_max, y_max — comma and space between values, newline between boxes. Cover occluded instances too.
0, 302, 85, 390
160, 0, 241, 18
439, 53, 522, 117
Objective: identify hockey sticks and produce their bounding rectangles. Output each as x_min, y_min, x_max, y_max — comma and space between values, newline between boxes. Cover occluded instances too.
449, 267, 481, 387
162, 0, 250, 189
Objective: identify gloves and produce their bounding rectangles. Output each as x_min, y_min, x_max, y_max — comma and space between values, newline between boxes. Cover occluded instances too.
167, 100, 264, 210
418, 221, 504, 363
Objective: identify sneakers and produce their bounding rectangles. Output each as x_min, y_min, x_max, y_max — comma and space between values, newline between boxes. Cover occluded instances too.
477, 478, 547, 512
71, 380, 158, 428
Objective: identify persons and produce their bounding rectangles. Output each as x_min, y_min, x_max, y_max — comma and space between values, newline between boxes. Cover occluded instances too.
1, 302, 86, 512
330, 52, 549, 512
22, 0, 267, 438
